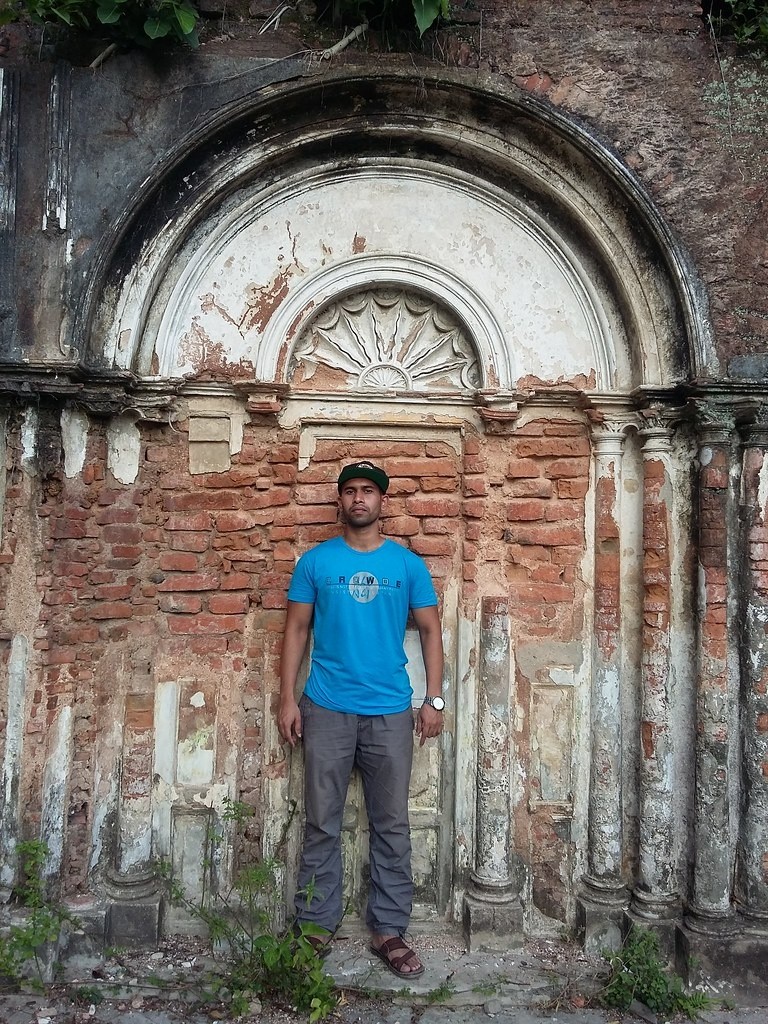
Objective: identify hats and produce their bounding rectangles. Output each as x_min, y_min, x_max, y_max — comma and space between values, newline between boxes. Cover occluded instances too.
337, 461, 390, 494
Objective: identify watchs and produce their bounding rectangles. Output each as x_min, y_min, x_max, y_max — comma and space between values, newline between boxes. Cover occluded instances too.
424, 696, 446, 711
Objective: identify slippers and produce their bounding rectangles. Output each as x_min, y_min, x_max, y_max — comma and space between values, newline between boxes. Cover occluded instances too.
370, 936, 425, 979
289, 937, 332, 972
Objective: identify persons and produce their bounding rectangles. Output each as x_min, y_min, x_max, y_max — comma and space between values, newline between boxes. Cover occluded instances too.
278, 460, 449, 979
